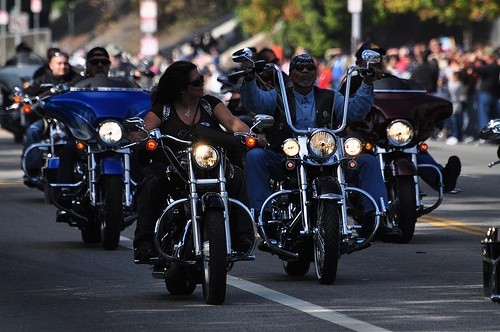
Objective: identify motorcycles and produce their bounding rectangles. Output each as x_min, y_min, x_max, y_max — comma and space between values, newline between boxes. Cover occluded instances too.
119, 111, 275, 306
477, 116, 500, 305
335, 64, 462, 245
228, 46, 387, 288
0, 46, 158, 251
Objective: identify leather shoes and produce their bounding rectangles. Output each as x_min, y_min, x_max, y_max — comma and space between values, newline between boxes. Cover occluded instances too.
258, 239, 279, 252
360, 215, 402, 240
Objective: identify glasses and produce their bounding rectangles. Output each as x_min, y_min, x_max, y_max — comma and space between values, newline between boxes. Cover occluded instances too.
55, 52, 68, 58
291, 64, 316, 72
88, 59, 110, 66
189, 75, 204, 87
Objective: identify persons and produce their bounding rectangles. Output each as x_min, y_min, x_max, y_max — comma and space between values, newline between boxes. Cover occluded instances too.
69, 41, 230, 106
126, 62, 268, 261
220, 48, 404, 243
4, 39, 80, 191
233, 35, 500, 146
340, 42, 461, 194
36, 47, 144, 224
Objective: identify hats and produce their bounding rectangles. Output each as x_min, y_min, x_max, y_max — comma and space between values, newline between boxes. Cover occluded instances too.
105, 44, 121, 57
87, 47, 109, 58
356, 42, 386, 60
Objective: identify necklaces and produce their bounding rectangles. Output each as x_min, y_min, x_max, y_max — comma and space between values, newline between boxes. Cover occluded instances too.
179, 101, 195, 118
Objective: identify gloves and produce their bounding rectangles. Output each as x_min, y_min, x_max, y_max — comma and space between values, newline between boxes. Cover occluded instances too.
243, 67, 257, 82
362, 68, 383, 84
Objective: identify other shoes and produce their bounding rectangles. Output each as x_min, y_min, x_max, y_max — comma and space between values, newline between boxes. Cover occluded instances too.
134, 246, 157, 262
442, 155, 461, 191
446, 136, 458, 145
